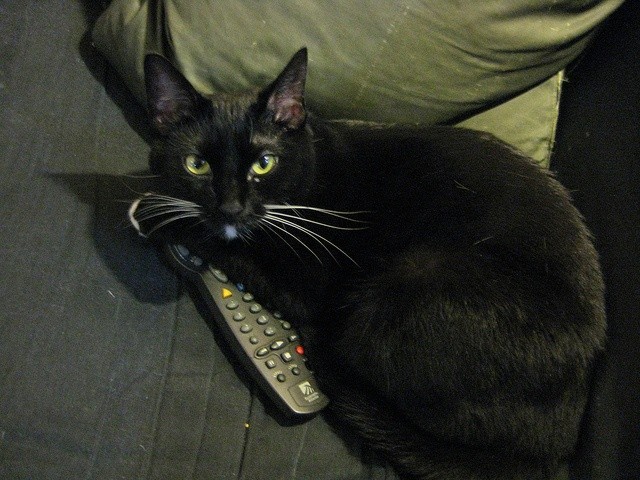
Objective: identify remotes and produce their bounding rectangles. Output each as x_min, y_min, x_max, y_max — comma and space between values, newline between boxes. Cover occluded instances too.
163, 241, 331, 420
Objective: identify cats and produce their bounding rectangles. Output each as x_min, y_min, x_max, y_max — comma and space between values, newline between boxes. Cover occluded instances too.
118, 46, 610, 480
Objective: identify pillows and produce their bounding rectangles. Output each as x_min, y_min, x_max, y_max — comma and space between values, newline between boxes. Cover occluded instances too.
92, 0, 630, 194
